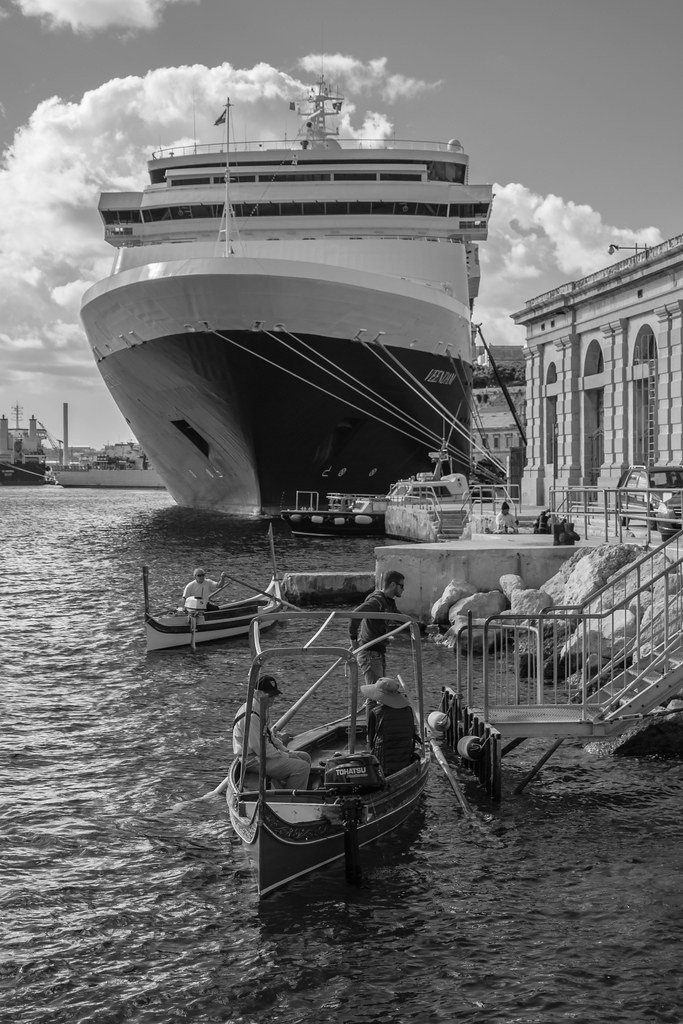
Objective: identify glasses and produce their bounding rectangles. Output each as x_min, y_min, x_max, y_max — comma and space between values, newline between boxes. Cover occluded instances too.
196, 574, 206, 577
398, 584, 404, 588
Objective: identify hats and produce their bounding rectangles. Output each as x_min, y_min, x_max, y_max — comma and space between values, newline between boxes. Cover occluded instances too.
502, 502, 510, 510
360, 677, 410, 709
253, 676, 282, 697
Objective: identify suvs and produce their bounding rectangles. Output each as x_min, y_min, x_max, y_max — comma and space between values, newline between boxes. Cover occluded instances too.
615, 465, 683, 532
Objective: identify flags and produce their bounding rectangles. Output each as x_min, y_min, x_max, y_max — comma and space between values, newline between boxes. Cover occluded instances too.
215, 108, 227, 126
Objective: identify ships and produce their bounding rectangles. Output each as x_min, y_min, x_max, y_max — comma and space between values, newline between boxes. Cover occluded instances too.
76, 63, 493, 519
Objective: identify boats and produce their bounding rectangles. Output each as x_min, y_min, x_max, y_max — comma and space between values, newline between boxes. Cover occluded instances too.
230, 603, 427, 922
140, 521, 286, 651
276, 400, 498, 539
49, 440, 168, 488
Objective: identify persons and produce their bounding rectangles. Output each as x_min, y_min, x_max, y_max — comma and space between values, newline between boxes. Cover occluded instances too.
182, 567, 227, 611
361, 676, 417, 778
346, 570, 439, 739
496, 502, 520, 535
231, 675, 313, 791
